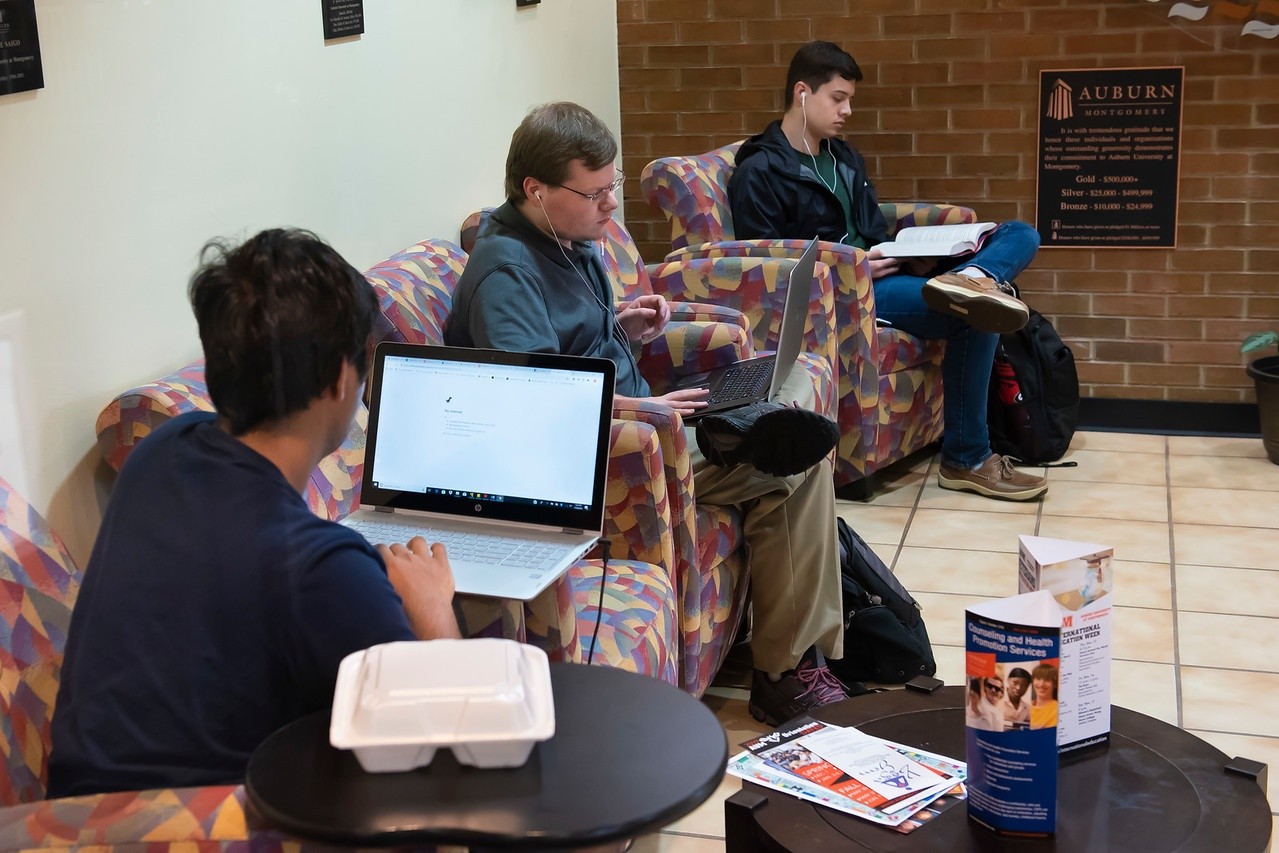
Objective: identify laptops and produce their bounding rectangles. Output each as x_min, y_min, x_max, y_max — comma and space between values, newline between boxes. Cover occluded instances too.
336, 340, 615, 600
669, 235, 820, 421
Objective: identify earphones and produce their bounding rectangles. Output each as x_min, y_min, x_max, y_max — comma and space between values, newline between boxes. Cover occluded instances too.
801, 91, 806, 107
534, 190, 541, 199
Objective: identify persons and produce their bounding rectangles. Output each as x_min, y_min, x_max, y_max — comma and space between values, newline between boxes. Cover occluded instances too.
965, 664, 1058, 732
727, 42, 1050, 501
445, 103, 848, 727
41, 225, 461, 796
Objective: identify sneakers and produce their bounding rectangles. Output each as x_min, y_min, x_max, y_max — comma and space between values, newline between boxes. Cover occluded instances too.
696, 401, 841, 478
922, 272, 1030, 334
748, 645, 851, 728
939, 453, 1048, 502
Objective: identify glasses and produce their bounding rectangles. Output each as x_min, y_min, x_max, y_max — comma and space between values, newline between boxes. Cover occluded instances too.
552, 169, 626, 206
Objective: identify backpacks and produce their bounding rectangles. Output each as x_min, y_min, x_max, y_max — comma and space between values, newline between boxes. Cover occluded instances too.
825, 518, 936, 695
988, 308, 1080, 467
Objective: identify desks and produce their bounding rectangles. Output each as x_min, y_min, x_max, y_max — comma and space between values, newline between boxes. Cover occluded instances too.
244, 666, 729, 853
725, 684, 1274, 853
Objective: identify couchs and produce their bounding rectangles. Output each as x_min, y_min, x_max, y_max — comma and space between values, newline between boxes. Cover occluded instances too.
0, 130, 978, 853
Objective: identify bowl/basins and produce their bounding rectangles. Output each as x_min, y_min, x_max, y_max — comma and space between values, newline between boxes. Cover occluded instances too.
330, 638, 554, 770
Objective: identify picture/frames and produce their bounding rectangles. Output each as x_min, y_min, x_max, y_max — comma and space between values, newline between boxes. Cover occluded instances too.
1033, 66, 1187, 249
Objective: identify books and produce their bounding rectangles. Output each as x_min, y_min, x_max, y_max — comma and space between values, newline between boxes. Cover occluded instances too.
870, 222, 1000, 259
